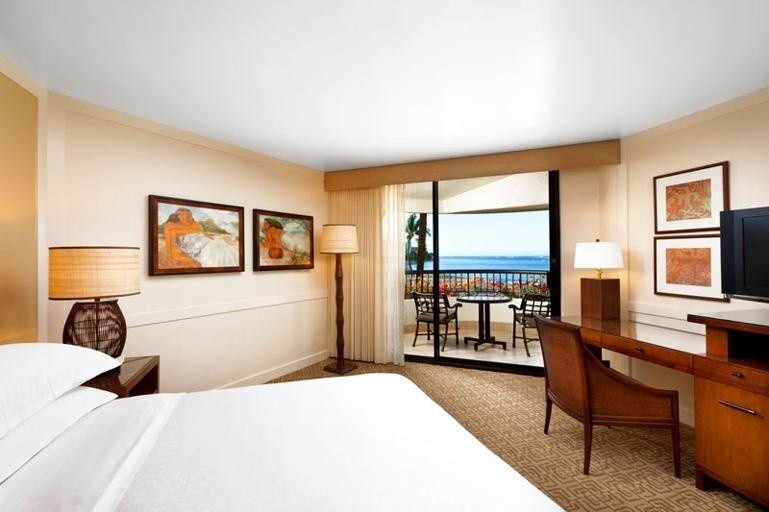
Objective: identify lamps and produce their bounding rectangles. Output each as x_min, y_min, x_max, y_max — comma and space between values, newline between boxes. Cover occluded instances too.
48, 246, 141, 376
573, 239, 625, 322
320, 224, 360, 376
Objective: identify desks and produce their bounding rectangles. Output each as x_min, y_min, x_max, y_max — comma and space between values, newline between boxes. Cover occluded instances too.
456, 290, 512, 351
555, 307, 768, 508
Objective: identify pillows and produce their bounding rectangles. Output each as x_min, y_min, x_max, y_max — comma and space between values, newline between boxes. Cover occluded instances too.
0, 342, 125, 484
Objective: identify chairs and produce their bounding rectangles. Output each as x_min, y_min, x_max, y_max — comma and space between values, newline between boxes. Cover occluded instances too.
412, 291, 463, 351
509, 294, 551, 358
533, 311, 681, 479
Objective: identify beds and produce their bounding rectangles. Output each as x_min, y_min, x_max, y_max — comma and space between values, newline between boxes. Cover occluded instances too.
0, 372, 568, 512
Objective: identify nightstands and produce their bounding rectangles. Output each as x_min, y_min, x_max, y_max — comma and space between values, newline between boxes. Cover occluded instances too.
83, 356, 161, 396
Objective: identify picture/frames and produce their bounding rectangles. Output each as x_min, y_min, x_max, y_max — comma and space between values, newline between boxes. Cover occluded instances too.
653, 159, 729, 303
149, 194, 313, 274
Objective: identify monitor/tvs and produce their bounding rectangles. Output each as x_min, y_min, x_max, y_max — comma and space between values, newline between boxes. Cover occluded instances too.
719, 206, 769, 297
